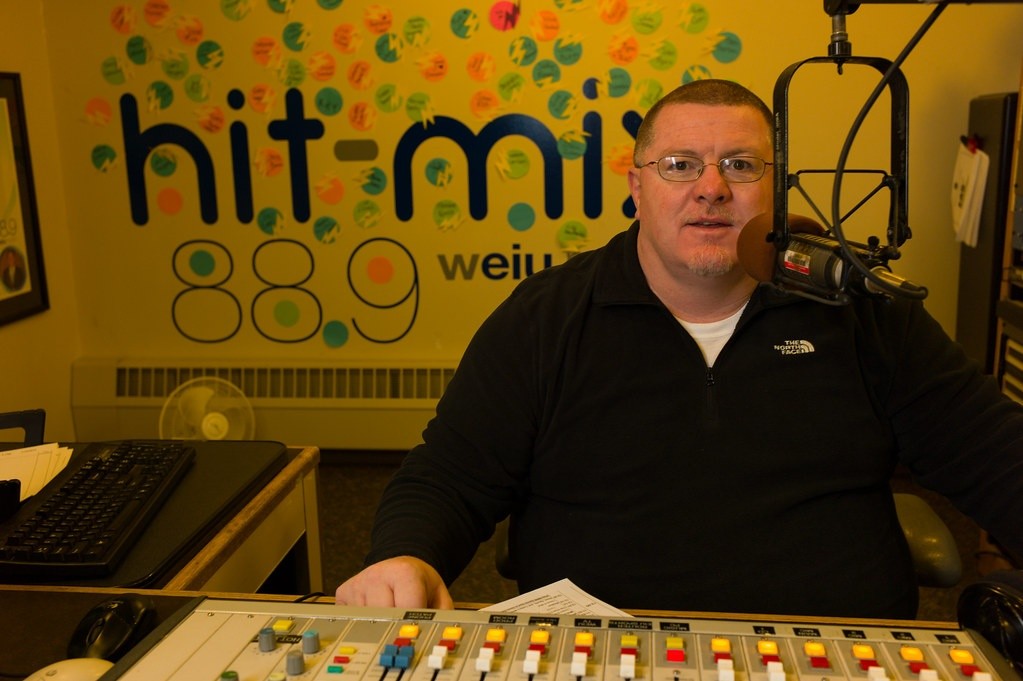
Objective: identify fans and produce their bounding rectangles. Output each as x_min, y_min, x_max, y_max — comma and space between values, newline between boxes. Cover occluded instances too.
156, 376, 259, 442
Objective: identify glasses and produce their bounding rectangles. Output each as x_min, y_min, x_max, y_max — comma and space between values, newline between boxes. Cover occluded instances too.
640, 156, 774, 183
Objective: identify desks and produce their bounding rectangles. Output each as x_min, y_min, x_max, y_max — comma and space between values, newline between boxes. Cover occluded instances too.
0, 446, 326, 596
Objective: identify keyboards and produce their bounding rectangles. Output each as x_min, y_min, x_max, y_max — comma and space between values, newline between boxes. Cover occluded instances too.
0, 439, 198, 583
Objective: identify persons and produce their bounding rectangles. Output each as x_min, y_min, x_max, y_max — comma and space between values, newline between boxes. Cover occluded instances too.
3, 250, 26, 290
336, 79, 1023, 619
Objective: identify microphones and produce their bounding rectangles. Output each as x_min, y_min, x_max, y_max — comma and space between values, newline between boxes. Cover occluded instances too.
737, 210, 928, 306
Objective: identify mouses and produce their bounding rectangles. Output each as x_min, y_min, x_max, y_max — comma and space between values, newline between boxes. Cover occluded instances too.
66, 594, 158, 665
23, 658, 113, 681
